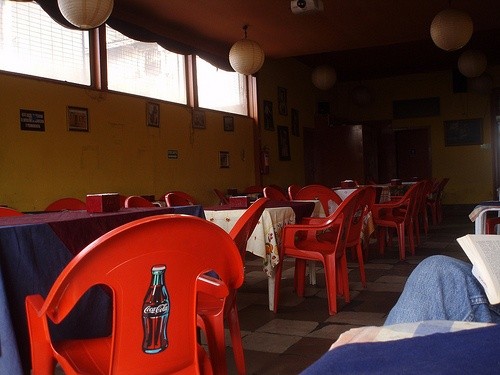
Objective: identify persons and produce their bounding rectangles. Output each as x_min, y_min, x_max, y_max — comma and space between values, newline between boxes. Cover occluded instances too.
384, 255, 500, 327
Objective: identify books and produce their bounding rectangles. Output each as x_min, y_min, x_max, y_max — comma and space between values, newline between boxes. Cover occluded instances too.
457, 234, 500, 304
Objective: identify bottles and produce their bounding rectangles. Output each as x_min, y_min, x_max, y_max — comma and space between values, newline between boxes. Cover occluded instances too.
496, 184, 500, 201
141, 264, 170, 354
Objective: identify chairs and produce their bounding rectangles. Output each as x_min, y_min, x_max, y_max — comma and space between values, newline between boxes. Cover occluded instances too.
0, 177, 451, 375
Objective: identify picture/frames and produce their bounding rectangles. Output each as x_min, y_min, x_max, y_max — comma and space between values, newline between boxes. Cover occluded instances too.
145, 101, 160, 128
264, 100, 275, 130
168, 150, 178, 159
65, 104, 88, 132
223, 115, 234, 132
291, 108, 299, 136
277, 86, 288, 116
192, 109, 206, 129
218, 151, 230, 168
444, 118, 484, 147
276, 124, 291, 161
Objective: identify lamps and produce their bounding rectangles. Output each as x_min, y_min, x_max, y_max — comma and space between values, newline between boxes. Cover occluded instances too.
431, 0, 474, 51
229, 26, 265, 75
456, 44, 488, 79
56, 0, 114, 30
311, 65, 336, 91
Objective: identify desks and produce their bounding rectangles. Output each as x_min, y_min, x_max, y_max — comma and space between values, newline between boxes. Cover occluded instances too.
199, 200, 320, 312
320, 184, 392, 260
1, 203, 207, 375
